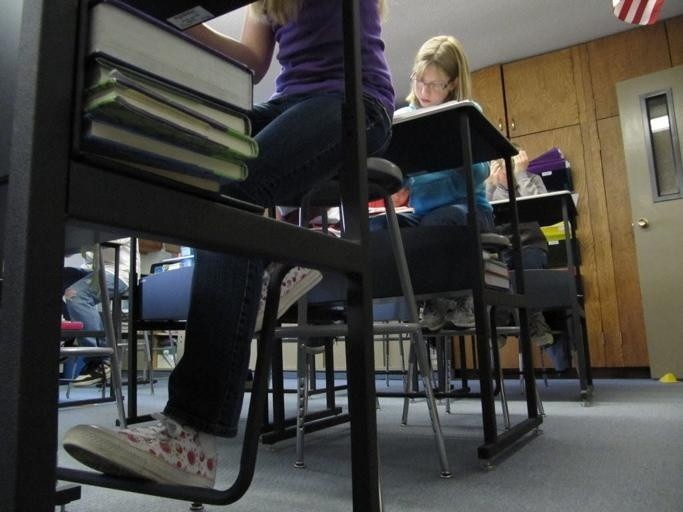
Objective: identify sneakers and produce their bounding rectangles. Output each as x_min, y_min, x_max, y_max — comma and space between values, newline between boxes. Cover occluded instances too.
445, 296, 478, 328
70, 363, 111, 388
252, 266, 323, 334
420, 298, 456, 332
61, 416, 218, 490
527, 311, 554, 348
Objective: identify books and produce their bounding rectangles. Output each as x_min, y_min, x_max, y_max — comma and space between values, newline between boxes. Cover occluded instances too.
72, 0, 260, 200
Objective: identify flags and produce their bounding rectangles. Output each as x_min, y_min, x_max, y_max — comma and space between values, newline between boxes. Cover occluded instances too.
609, 0, 665, 24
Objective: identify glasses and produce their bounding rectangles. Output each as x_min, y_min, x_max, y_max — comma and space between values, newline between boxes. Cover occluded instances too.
414, 79, 456, 92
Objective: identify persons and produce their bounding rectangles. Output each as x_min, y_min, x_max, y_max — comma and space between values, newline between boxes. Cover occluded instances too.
60, 144, 560, 389
369, 32, 496, 335
65, 1, 400, 489
